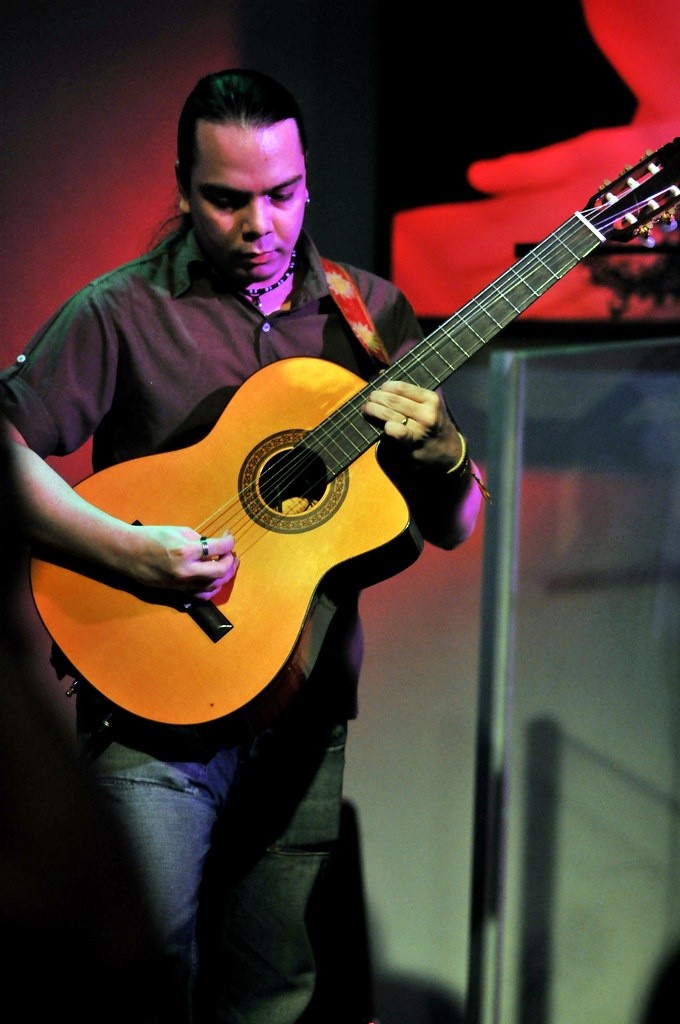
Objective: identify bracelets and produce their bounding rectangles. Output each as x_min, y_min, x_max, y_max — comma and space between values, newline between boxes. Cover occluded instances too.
447, 431, 494, 507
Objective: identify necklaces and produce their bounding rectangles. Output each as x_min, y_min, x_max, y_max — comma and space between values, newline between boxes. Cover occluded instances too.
240, 250, 297, 308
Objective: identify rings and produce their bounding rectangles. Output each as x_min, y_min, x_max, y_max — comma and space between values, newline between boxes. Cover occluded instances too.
401, 416, 408, 425
201, 537, 209, 560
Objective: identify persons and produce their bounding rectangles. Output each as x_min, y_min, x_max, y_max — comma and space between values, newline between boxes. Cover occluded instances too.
0, 67, 483, 1024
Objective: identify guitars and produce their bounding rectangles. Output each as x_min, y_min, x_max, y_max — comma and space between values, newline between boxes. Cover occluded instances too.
30, 134, 680, 763
